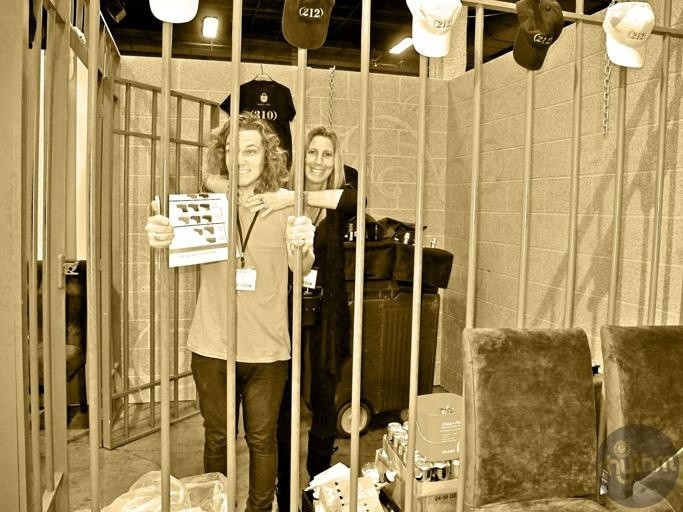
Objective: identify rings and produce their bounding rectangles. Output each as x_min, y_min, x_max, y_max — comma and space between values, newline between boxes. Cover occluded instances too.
260, 199, 263, 204
270, 209, 274, 211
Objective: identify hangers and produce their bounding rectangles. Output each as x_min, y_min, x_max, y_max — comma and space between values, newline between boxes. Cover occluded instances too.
252, 63, 274, 82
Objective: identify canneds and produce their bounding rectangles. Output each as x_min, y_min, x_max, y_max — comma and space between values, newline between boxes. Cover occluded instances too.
387, 421, 460, 483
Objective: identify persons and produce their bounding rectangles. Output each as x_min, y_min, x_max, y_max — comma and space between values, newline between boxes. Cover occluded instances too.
205, 125, 366, 512
147, 115, 317, 512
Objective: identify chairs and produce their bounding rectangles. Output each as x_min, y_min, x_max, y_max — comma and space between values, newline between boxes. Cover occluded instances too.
458, 323, 615, 512
595, 320, 682, 512
23, 258, 89, 428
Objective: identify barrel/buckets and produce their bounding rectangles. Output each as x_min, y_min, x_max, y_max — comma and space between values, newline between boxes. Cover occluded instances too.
415, 391, 464, 464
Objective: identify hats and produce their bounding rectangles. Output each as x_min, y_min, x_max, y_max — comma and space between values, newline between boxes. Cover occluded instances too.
602, 2, 655, 69
281, 0, 336, 52
513, 1, 564, 71
148, 0, 199, 26
405, 0, 463, 58
